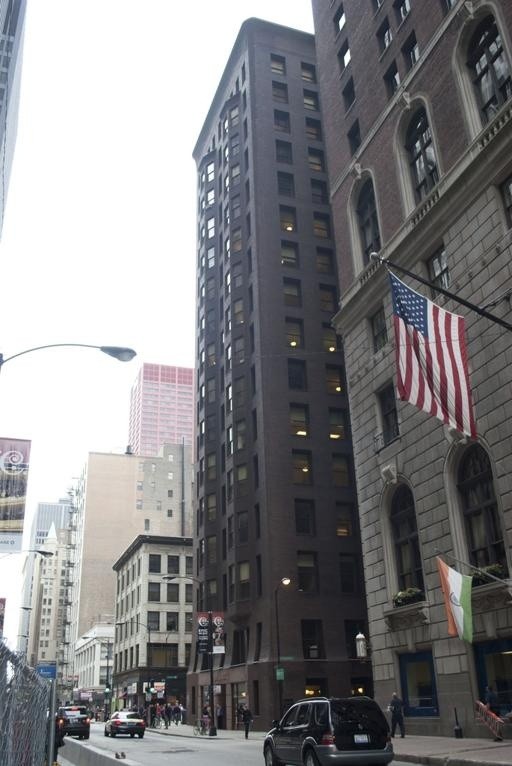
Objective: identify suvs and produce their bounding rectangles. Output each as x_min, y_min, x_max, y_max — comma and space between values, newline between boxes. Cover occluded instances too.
58, 706, 91, 740
263, 695, 394, 766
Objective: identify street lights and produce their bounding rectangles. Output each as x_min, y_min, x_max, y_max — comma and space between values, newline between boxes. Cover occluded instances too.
1, 548, 55, 563
81, 636, 110, 721
162, 575, 217, 737
164, 618, 193, 707
115, 621, 151, 689
274, 577, 291, 719
0, 340, 139, 365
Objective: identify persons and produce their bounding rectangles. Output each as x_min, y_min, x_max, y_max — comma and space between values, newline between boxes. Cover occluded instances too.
45, 709, 68, 763
242, 704, 254, 739
388, 691, 406, 738
45, 699, 226, 730
483, 685, 500, 728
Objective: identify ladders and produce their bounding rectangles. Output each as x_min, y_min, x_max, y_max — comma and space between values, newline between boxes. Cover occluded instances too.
474, 700, 504, 739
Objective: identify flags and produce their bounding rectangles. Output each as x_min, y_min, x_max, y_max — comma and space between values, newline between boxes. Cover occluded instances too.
436, 556, 474, 644
385, 263, 479, 441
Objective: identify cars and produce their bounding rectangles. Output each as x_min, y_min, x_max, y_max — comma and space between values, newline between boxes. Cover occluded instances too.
105, 711, 145, 739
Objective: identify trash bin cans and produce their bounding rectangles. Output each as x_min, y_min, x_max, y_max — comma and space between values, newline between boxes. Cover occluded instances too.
97, 709, 105, 722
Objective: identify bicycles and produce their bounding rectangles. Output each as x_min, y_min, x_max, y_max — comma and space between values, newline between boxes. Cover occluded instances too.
193, 719, 212, 737
151, 716, 163, 729
